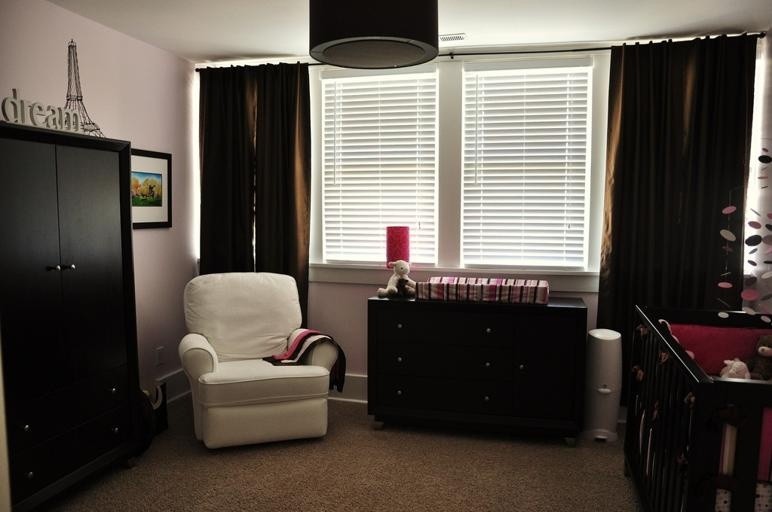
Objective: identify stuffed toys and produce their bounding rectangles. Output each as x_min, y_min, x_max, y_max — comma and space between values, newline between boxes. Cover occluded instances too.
396, 279, 416, 298
376, 259, 416, 297
719, 358, 751, 380
657, 318, 694, 362
747, 334, 772, 380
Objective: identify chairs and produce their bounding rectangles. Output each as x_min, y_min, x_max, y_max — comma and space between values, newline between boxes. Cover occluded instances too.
179, 272, 339, 453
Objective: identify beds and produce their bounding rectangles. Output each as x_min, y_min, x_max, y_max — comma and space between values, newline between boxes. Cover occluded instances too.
622, 304, 772, 512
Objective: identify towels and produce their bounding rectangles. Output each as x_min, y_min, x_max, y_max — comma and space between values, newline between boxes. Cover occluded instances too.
263, 328, 346, 393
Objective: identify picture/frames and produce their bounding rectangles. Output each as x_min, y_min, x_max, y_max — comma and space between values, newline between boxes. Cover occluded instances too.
131, 148, 172, 229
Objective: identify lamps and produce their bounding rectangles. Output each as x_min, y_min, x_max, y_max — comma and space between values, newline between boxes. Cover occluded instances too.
309, 0, 438, 69
386, 226, 409, 269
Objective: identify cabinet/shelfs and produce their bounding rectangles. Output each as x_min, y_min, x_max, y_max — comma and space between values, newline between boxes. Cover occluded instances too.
1, 121, 142, 512
367, 295, 588, 446
